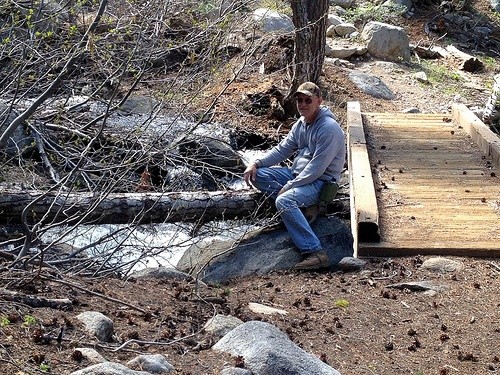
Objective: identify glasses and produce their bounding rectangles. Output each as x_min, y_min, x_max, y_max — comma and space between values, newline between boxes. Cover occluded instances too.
297, 96, 319, 104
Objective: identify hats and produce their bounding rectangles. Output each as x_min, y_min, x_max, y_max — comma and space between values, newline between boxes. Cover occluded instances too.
293, 82, 321, 97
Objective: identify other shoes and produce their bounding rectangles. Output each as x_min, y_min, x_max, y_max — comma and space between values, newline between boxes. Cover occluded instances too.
304, 205, 317, 225
295, 250, 331, 269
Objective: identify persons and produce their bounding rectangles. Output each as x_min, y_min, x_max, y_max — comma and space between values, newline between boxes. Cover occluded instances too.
244, 82, 346, 270
